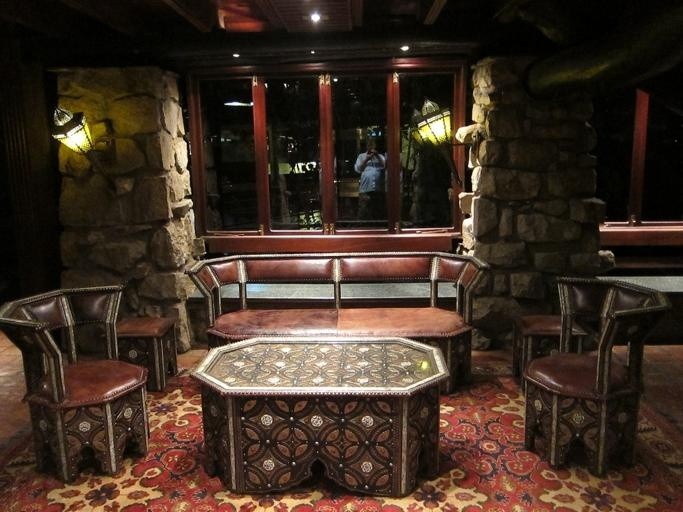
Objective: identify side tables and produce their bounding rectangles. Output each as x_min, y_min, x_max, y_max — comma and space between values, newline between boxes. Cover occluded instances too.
106, 315, 180, 393
510, 314, 588, 396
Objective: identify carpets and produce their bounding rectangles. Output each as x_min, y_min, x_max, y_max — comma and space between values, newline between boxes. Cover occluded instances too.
0, 350, 681, 512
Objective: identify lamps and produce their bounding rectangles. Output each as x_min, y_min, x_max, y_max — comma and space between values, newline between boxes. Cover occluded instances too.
48, 105, 118, 191
413, 96, 473, 188
409, 106, 424, 150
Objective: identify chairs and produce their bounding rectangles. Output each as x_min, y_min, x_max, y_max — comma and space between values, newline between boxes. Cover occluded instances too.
518, 273, 673, 478
0, 283, 152, 480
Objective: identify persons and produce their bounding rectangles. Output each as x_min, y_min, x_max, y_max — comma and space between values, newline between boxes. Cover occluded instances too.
354, 137, 388, 228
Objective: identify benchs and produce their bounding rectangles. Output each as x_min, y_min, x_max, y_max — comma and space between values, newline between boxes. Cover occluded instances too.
184, 248, 492, 395
